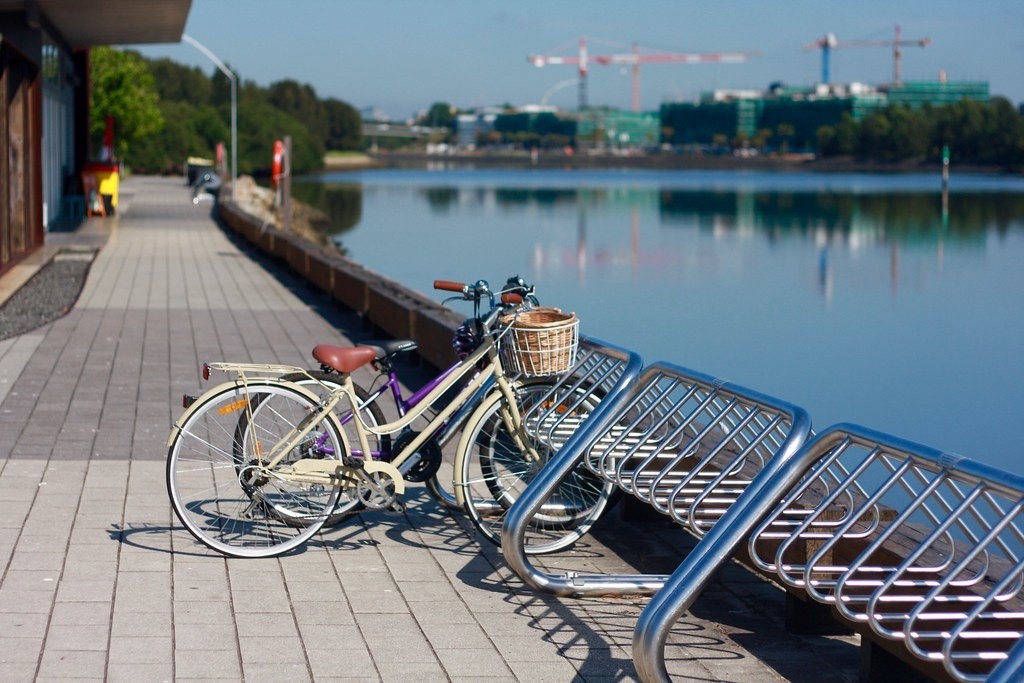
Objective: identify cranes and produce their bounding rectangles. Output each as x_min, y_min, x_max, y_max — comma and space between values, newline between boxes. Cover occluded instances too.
803, 24, 929, 94
530, 36, 748, 116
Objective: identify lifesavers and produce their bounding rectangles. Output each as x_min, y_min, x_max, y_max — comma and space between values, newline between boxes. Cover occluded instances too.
271, 139, 283, 187
216, 142, 224, 164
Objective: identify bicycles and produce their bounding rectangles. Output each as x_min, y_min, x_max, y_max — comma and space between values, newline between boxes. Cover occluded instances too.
165, 277, 615, 557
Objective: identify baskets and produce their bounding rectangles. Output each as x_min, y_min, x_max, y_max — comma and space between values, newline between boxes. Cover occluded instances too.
494, 306, 580, 377
